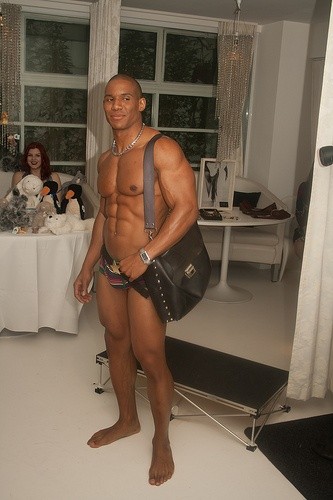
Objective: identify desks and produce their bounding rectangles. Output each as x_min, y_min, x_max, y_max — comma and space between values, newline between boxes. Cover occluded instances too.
196, 202, 295, 304
0, 225, 95, 338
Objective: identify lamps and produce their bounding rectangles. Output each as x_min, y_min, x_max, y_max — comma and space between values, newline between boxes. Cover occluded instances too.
0, 0, 22, 155
213, 0, 257, 164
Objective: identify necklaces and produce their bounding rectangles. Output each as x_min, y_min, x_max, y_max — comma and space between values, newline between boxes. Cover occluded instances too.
112, 123, 146, 155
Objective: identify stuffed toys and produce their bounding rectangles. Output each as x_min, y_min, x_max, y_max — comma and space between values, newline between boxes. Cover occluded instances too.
3, 175, 89, 236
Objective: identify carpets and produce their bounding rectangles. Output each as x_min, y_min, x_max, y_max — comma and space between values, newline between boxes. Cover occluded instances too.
243, 413, 333, 500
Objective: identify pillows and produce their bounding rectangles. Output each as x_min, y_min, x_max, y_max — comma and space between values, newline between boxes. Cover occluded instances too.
232, 190, 262, 208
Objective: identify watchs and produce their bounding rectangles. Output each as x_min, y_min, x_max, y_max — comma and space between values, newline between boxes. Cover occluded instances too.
138, 248, 151, 266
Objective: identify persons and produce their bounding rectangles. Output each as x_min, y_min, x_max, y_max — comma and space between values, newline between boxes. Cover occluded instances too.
73, 74, 200, 486
12, 142, 62, 203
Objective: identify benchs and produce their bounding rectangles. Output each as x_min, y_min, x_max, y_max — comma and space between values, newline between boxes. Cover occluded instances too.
193, 171, 291, 282
94, 334, 292, 452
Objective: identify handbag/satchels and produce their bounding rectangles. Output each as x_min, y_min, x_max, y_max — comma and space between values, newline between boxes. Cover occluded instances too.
142, 219, 212, 322
